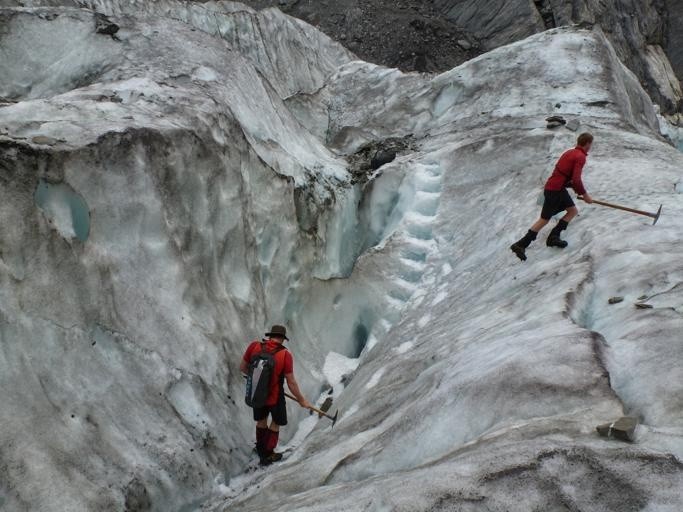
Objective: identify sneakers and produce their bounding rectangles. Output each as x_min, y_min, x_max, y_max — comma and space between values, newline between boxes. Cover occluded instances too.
510, 243, 527, 261
546, 238, 567, 247
259, 453, 282, 464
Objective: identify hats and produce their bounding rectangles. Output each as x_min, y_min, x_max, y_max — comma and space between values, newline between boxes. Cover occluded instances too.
265, 326, 289, 341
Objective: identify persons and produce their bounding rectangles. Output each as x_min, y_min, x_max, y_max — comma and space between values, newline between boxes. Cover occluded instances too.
238, 325, 310, 467
508, 132, 593, 261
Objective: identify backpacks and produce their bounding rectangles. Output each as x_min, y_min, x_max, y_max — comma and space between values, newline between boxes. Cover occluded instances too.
245, 343, 287, 408
